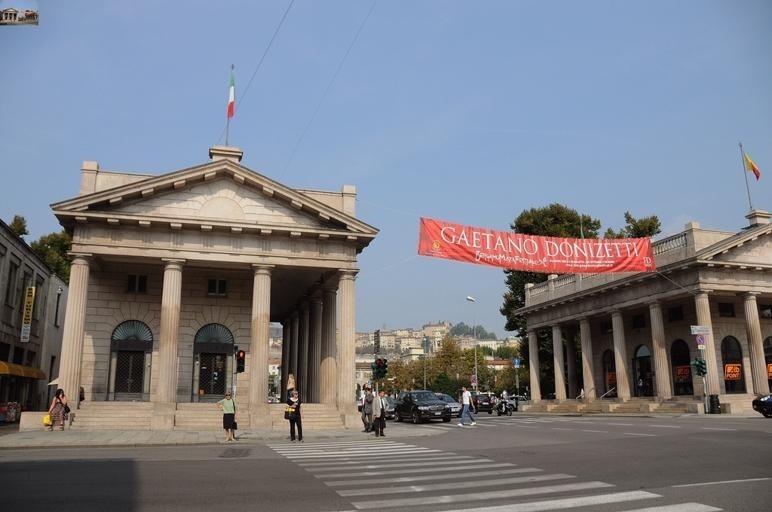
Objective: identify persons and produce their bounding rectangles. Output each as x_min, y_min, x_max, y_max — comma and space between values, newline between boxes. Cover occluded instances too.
360, 386, 374, 433
47, 388, 68, 432
14, 402, 22, 428
286, 390, 305, 443
456, 384, 476, 426
372, 390, 389, 437
287, 373, 296, 400
216, 390, 238, 443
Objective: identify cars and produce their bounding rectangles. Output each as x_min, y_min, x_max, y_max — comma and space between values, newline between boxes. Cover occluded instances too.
750, 393, 772, 418
355, 387, 462, 423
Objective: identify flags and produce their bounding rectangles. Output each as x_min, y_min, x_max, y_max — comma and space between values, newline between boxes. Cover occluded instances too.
739, 142, 760, 183
227, 63, 237, 119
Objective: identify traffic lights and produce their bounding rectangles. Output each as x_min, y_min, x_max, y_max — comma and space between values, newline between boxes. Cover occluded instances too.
694, 355, 708, 378
371, 357, 388, 381
237, 349, 246, 373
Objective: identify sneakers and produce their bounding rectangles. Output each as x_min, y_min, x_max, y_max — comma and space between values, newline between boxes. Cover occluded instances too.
470, 422, 476, 426
362, 427, 385, 436
457, 422, 464, 428
293, 440, 296, 442
299, 440, 304, 443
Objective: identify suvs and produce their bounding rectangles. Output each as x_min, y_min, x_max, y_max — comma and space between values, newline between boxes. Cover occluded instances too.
504, 395, 528, 411
467, 394, 493, 414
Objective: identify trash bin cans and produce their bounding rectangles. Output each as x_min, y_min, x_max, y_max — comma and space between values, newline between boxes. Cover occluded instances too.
709, 394, 721, 414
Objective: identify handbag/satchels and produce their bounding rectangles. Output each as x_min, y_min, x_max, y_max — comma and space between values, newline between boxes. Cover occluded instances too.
43, 415, 51, 426
65, 406, 70, 412
234, 422, 237, 430
290, 414, 299, 422
284, 405, 295, 419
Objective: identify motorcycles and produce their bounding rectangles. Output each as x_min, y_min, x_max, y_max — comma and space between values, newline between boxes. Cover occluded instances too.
496, 398, 513, 416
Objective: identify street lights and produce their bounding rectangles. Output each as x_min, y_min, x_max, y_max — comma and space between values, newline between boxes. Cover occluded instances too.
467, 293, 477, 405
422, 337, 427, 389
